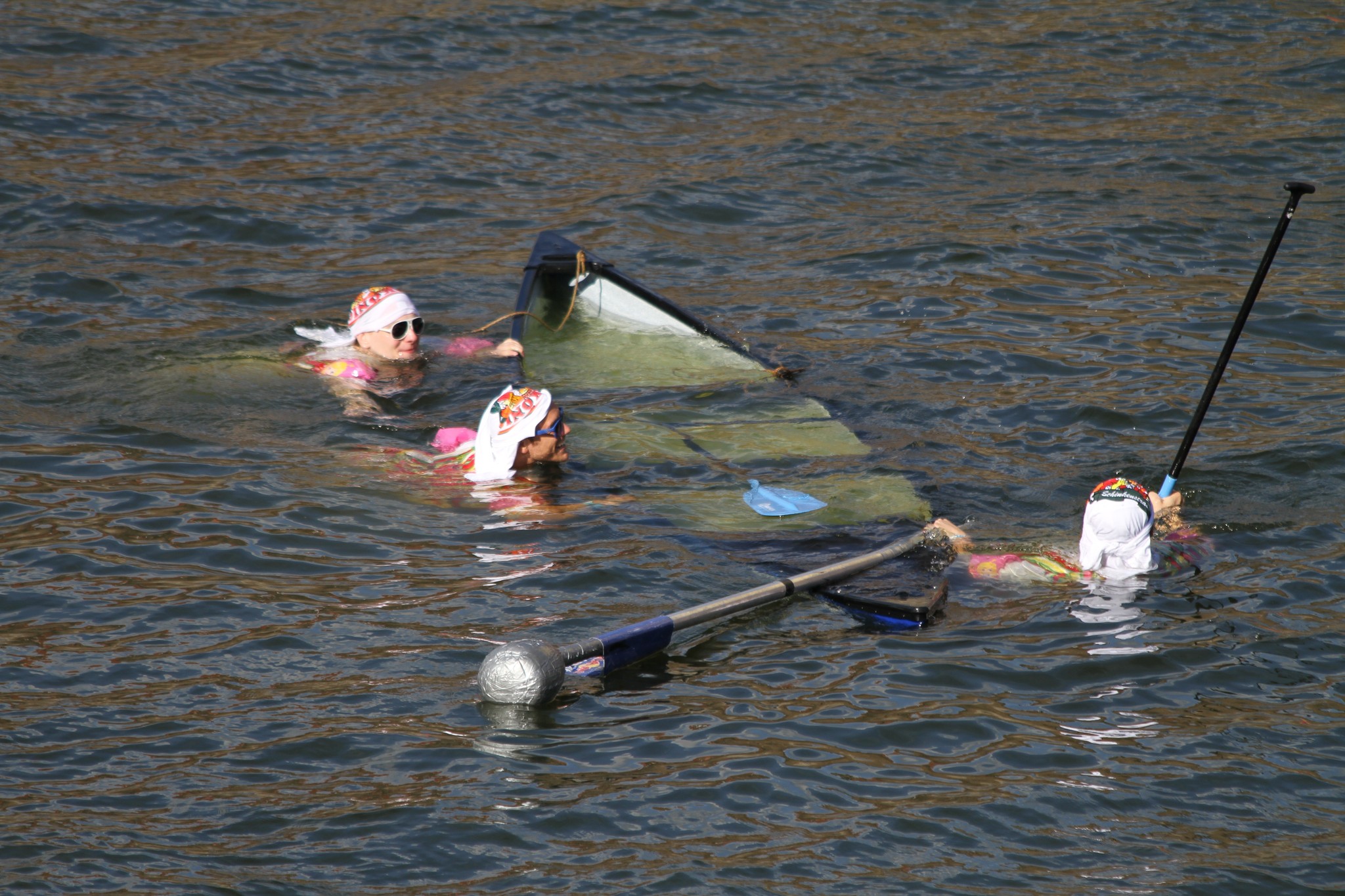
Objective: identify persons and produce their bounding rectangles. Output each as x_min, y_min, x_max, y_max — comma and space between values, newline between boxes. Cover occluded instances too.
928, 477, 1216, 589
301, 282, 523, 424
437, 383, 639, 526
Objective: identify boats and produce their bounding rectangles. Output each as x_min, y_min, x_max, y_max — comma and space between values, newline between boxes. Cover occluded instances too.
506, 229, 952, 633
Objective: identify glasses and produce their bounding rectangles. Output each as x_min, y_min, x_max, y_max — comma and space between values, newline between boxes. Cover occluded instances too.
535, 407, 564, 438
379, 317, 424, 340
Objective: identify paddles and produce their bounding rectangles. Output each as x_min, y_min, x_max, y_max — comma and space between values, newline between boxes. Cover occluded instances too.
1157, 178, 1318, 501
650, 420, 828, 516
